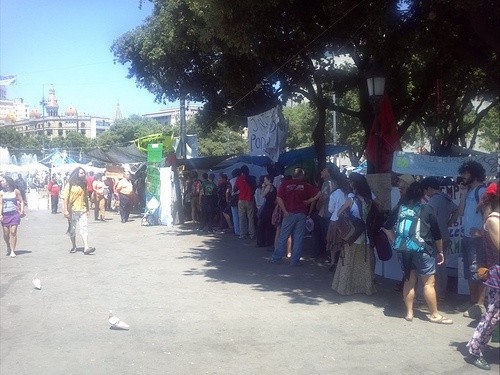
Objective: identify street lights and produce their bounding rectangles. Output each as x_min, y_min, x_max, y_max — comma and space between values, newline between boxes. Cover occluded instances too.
366, 58, 386, 172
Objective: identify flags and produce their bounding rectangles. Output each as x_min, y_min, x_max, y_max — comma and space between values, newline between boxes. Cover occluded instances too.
363, 95, 403, 185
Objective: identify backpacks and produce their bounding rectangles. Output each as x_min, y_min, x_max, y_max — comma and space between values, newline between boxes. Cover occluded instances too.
391, 200, 428, 254
335, 210, 364, 244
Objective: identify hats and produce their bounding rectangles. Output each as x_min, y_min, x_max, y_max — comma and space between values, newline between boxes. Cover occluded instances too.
398, 174, 416, 185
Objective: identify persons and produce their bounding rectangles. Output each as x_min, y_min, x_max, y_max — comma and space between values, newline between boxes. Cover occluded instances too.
0, 167, 146, 258
182, 161, 492, 323
466, 179, 500, 370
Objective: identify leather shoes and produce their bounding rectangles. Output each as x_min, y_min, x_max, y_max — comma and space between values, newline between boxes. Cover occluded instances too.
469, 354, 490, 369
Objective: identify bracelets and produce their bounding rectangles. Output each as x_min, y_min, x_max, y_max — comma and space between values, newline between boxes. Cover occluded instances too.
436, 251, 444, 253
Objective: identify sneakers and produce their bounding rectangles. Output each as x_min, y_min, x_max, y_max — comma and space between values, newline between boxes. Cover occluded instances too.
464, 304, 485, 317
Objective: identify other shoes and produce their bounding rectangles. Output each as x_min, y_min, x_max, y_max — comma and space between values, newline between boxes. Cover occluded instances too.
11, 252, 17, 258
84, 246, 96, 255
70, 247, 77, 253
6, 250, 12, 255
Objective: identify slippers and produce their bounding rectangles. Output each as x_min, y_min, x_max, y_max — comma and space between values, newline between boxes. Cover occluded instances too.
430, 313, 454, 324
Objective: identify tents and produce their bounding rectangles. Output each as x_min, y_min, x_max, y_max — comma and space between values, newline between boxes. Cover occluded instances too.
0, 143, 145, 164
